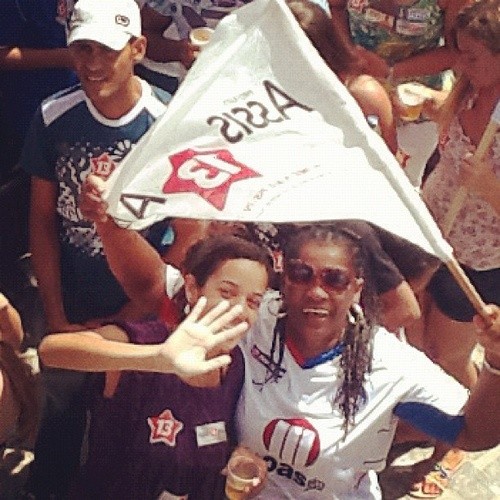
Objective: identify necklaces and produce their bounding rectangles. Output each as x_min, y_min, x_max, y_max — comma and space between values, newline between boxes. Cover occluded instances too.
464, 88, 482, 111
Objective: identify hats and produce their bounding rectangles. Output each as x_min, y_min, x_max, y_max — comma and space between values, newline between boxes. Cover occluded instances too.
67, 1, 143, 52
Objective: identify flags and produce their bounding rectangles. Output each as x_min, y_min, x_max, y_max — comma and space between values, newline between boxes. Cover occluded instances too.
98, 0, 453, 262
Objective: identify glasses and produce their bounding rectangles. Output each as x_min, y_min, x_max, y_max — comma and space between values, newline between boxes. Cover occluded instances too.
284, 261, 358, 291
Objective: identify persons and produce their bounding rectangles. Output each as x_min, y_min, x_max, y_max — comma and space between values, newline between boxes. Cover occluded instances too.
0, 0, 444, 349
378, 0, 500, 500
17, 0, 212, 500
0, 293, 41, 500
75, 173, 500, 500
326, 0, 473, 188
37, 234, 273, 500
285, 0, 399, 159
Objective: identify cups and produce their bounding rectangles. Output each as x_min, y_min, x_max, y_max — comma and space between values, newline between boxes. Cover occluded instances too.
189, 26, 214, 58
225, 456, 260, 500
398, 82, 425, 122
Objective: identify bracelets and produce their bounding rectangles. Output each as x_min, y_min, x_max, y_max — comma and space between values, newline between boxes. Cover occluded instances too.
482, 356, 500, 376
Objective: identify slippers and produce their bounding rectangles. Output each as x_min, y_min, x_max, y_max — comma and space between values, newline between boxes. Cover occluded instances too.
410, 454, 465, 498
